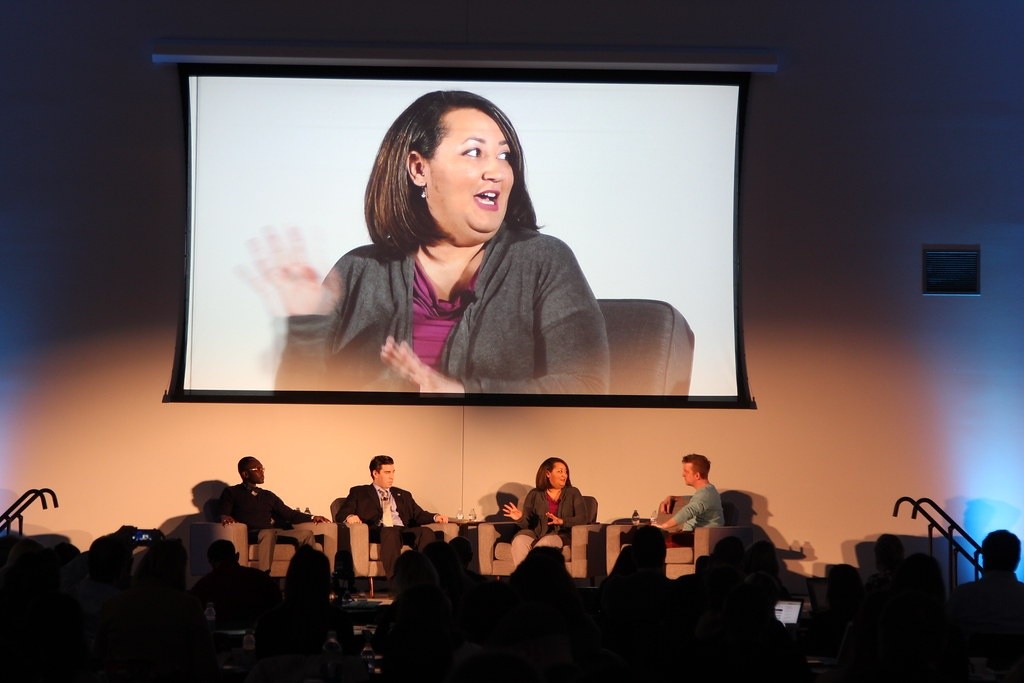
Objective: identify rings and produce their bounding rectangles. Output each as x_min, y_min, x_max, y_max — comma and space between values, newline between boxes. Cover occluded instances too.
224, 520, 226, 522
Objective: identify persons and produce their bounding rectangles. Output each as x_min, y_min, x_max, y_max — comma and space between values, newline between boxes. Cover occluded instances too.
335, 455, 449, 582
1, 529, 1024, 683
202, 456, 332, 575
503, 457, 587, 570
249, 86, 615, 396
651, 454, 725, 531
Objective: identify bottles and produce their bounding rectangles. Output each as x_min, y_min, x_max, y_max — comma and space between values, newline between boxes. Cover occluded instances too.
342, 590, 352, 604
469, 508, 476, 522
323, 631, 342, 681
304, 508, 311, 515
204, 603, 216, 632
243, 630, 256, 668
650, 511, 659, 527
631, 510, 640, 525
361, 639, 376, 673
456, 508, 464, 521
358, 591, 367, 602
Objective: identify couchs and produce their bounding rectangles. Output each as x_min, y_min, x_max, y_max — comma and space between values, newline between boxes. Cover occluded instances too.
330, 498, 459, 598
190, 500, 337, 578
478, 496, 604, 588
606, 495, 753, 580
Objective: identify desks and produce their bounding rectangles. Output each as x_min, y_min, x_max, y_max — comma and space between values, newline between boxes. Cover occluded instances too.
213, 624, 381, 681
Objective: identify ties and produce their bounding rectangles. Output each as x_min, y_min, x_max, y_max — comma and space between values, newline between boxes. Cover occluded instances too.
379, 489, 393, 528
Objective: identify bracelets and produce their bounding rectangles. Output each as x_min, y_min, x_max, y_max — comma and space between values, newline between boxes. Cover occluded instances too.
311, 516, 314, 520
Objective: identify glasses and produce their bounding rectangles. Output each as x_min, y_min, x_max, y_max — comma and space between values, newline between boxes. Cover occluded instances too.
244, 467, 265, 472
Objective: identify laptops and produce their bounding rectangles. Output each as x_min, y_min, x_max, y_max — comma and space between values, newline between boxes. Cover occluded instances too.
774, 598, 805, 627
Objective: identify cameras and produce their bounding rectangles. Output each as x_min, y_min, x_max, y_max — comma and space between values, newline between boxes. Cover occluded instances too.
129, 528, 161, 546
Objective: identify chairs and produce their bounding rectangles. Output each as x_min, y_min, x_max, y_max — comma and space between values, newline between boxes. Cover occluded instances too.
594, 297, 695, 396
241, 654, 371, 683
